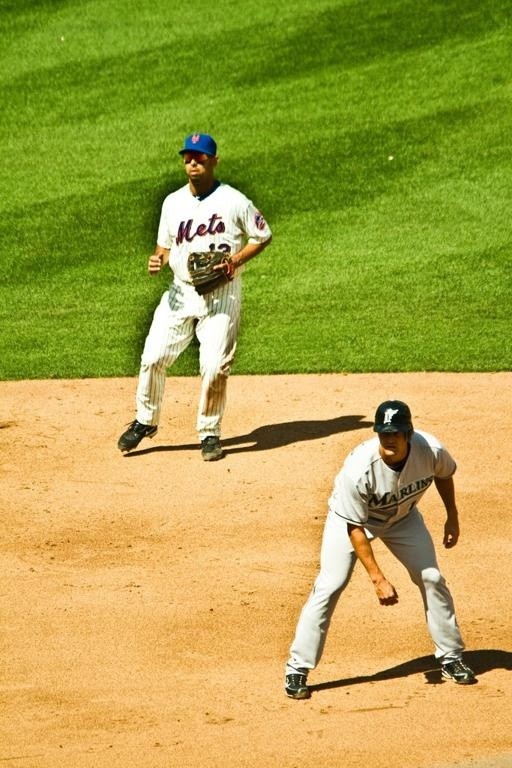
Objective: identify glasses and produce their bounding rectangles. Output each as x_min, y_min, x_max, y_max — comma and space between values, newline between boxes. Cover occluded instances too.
183, 152, 208, 162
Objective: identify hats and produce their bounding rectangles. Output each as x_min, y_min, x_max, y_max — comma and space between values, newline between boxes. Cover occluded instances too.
179, 134, 216, 157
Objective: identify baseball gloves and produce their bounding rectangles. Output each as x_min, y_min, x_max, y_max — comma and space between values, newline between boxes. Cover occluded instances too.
187, 252, 235, 296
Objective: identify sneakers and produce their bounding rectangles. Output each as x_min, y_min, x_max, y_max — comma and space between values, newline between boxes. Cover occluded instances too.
199, 436, 225, 461
441, 660, 475, 685
118, 419, 157, 452
284, 674, 311, 699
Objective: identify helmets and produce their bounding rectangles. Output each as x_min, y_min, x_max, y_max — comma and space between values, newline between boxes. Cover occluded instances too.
374, 400, 413, 434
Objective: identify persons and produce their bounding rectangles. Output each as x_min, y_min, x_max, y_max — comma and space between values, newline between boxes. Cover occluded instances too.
118, 132, 273, 461
286, 400, 476, 698
